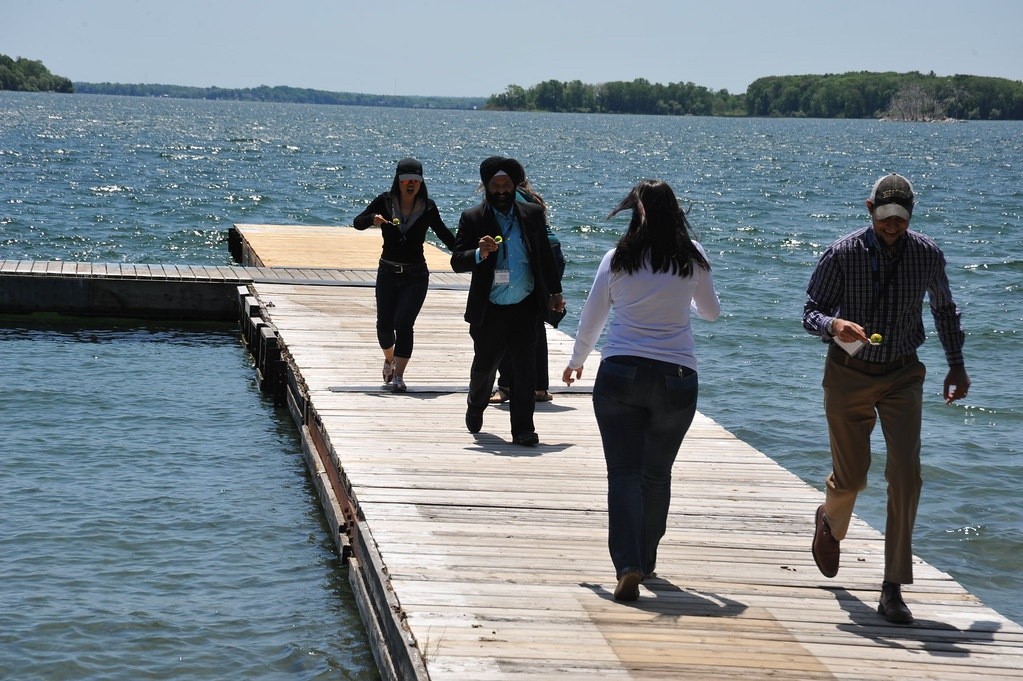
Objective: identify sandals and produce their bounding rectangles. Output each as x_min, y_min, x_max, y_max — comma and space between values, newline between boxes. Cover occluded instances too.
488, 386, 511, 403
534, 389, 552, 401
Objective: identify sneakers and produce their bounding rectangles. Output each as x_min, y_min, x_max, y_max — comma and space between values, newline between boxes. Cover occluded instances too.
391, 373, 406, 392
382, 359, 395, 383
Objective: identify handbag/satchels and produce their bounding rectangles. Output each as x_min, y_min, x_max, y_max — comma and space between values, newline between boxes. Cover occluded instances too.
545, 225, 567, 281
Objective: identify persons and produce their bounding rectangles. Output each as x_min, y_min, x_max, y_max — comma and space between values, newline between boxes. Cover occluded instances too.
801, 173, 971, 624
451, 156, 565, 445
353, 158, 455, 394
478, 179, 565, 402
562, 180, 721, 599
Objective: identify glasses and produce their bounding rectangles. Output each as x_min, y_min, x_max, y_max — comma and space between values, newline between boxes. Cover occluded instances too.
400, 180, 419, 186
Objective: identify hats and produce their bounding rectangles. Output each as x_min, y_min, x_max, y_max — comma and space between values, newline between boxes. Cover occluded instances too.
479, 155, 526, 186
396, 158, 423, 183
870, 174, 915, 220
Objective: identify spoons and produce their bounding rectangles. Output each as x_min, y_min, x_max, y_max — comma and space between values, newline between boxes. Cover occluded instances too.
388, 221, 400, 225
494, 242, 503, 244
867, 338, 881, 346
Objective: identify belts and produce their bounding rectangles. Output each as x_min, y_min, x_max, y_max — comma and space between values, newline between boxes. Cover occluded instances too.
828, 344, 918, 378
379, 259, 426, 274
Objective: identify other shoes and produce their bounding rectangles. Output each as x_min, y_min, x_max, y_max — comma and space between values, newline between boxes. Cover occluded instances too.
614, 570, 642, 602
642, 572, 657, 582
513, 431, 539, 445
466, 405, 484, 433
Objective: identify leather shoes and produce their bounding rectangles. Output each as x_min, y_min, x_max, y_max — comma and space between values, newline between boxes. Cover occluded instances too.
878, 580, 914, 623
812, 505, 841, 578
